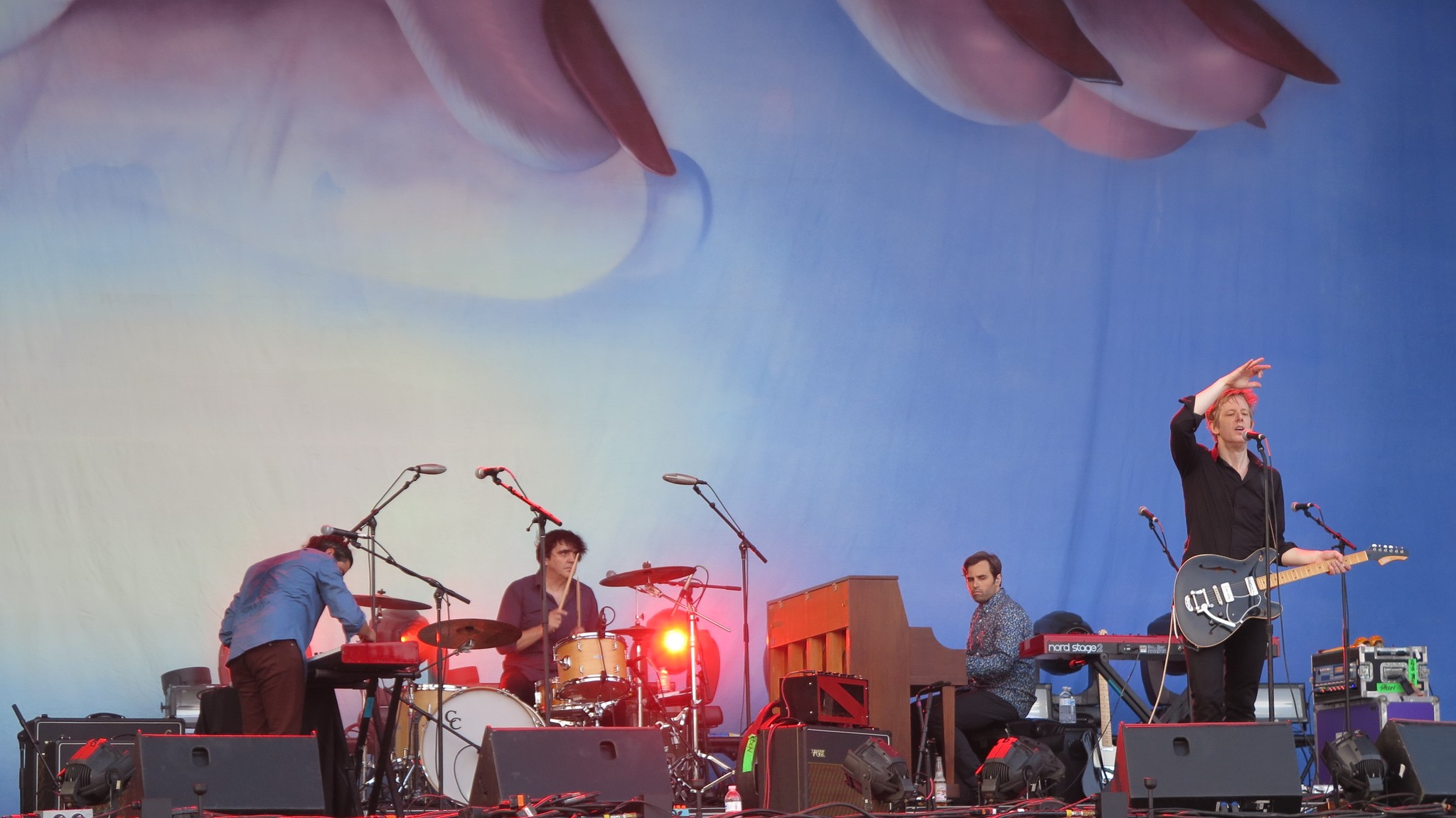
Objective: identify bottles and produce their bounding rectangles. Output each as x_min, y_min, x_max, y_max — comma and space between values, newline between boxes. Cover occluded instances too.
1059, 686, 1072, 724
1067, 687, 1077, 724
933, 756, 947, 807
724, 786, 742, 818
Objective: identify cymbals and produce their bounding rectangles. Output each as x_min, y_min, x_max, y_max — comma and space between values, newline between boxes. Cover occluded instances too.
417, 618, 522, 650
605, 624, 660, 637
600, 566, 697, 587
352, 594, 432, 610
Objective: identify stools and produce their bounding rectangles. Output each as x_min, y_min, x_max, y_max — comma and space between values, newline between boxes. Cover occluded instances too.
1005, 719, 1065, 742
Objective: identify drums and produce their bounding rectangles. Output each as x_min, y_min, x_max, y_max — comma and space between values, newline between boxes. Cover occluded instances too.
390, 684, 470, 772
553, 632, 630, 703
420, 687, 546, 807
533, 677, 613, 719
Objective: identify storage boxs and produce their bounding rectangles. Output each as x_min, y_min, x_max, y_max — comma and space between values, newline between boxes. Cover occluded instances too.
1316, 697, 1440, 789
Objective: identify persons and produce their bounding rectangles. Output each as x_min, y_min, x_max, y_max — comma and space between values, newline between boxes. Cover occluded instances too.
219, 534, 378, 738
1170, 357, 1351, 723
910, 551, 1037, 805
495, 529, 600, 707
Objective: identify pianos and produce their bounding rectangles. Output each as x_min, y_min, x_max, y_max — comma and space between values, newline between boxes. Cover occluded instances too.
1018, 631, 1280, 723
307, 640, 420, 818
766, 575, 968, 799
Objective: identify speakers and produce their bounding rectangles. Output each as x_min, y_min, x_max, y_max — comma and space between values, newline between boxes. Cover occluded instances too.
1376, 718, 1456, 808
756, 676, 892, 818
467, 724, 675, 818
1109, 719, 1304, 811
17, 714, 327, 818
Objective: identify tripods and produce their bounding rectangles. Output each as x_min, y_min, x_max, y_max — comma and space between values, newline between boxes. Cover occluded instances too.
644, 574, 735, 795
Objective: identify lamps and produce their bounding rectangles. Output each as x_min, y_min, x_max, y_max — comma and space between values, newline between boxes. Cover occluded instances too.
60, 737, 135, 810
975, 734, 1064, 804
1321, 729, 1390, 801
841, 738, 913, 804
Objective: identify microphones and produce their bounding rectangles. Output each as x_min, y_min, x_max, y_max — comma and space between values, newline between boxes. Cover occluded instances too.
606, 570, 648, 593
1242, 428, 1266, 441
1138, 507, 1159, 523
662, 472, 707, 485
320, 525, 371, 539
1291, 502, 1314, 512
407, 463, 447, 475
593, 608, 605, 630
670, 573, 695, 617
474, 466, 505, 479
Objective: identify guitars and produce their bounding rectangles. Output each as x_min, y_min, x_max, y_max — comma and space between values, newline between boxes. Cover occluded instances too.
1173, 544, 1409, 648
1082, 629, 1117, 800
